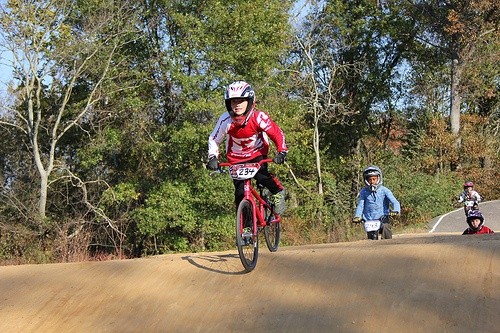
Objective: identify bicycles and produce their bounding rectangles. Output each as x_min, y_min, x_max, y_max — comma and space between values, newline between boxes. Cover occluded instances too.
217, 158, 284, 271
351, 213, 399, 241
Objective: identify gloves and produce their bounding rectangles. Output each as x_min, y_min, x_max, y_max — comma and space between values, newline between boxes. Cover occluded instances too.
391, 211, 399, 217
206, 158, 218, 171
353, 217, 361, 223
273, 153, 284, 164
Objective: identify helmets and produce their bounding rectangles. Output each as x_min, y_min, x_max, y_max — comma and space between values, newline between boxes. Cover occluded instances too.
363, 166, 383, 192
225, 82, 255, 126
464, 182, 474, 194
466, 211, 485, 231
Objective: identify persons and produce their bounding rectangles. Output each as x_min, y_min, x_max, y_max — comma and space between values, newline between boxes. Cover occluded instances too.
353, 165, 400, 239
457, 182, 481, 215
462, 210, 494, 235
207, 80, 288, 246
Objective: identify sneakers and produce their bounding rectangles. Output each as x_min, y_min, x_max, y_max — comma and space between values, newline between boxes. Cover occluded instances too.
241, 227, 252, 244
269, 190, 286, 213
